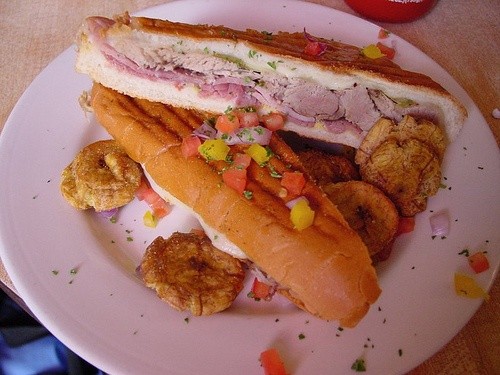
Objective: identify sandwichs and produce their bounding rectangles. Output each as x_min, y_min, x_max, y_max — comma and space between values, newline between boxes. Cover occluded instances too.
74, 9, 468, 150
88, 79, 383, 328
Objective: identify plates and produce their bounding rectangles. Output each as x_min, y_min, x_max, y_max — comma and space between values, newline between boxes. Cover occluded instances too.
0, 1, 500, 374
